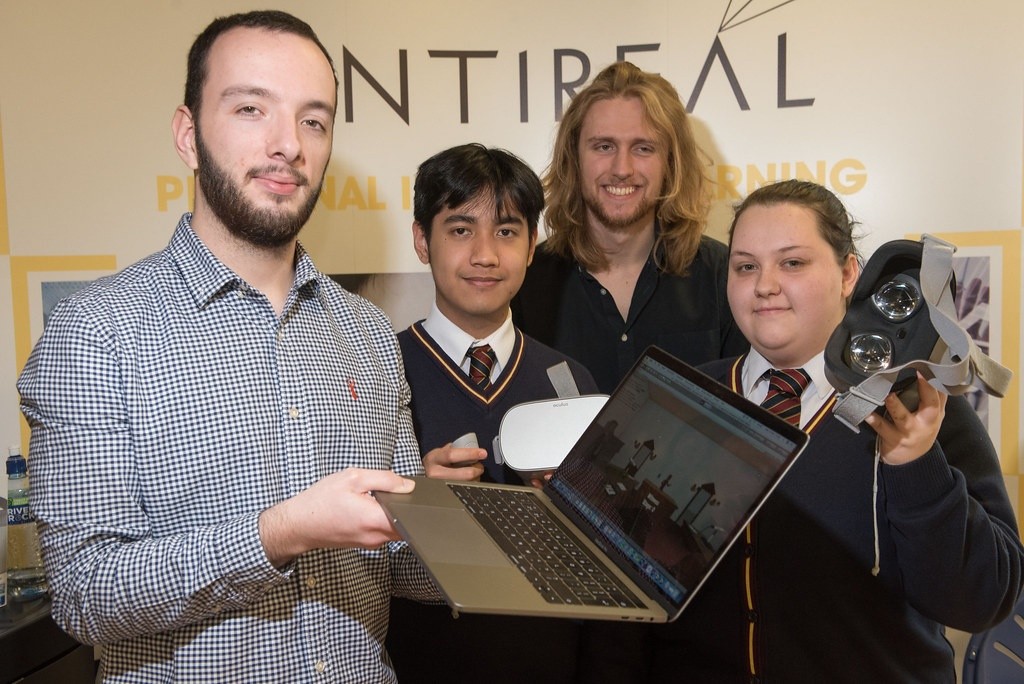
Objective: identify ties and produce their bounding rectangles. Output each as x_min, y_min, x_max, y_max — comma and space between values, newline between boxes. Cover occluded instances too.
465, 344, 496, 394
759, 368, 812, 429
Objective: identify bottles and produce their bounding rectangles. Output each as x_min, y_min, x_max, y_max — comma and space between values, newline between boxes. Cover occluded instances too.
6, 455, 48, 602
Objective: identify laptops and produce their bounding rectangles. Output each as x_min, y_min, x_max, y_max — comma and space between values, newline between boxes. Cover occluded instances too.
371, 344, 810, 624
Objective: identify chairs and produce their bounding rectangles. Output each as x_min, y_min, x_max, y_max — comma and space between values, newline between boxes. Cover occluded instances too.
962, 602, 1024, 684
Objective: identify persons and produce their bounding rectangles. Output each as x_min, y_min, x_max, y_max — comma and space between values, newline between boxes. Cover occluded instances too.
674, 180, 1024, 684
394, 141, 651, 684
504, 61, 751, 395
17, 11, 427, 684
